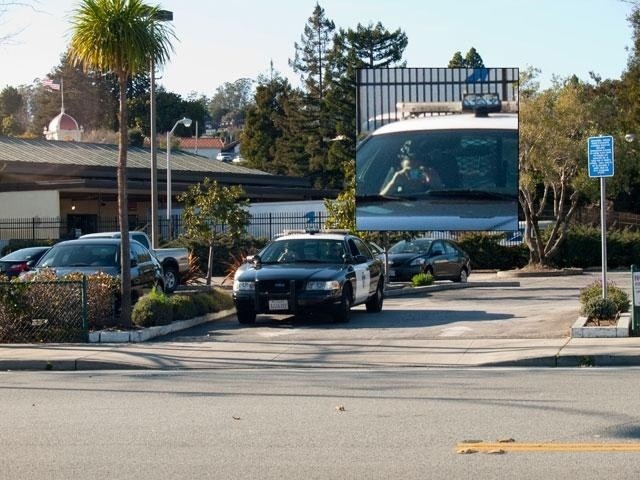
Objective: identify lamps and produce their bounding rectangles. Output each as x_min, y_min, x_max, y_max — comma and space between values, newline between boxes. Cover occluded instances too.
72, 202, 77, 212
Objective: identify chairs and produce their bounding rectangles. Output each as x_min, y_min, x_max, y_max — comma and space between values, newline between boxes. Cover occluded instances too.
429, 152, 461, 188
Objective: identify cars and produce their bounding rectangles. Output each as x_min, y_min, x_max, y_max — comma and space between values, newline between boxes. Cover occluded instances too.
232, 229, 472, 324
216, 153, 233, 162
0, 231, 190, 305
355, 94, 519, 230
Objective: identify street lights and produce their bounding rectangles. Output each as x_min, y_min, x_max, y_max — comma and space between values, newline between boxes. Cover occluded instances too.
151, 9, 173, 249
166, 117, 192, 220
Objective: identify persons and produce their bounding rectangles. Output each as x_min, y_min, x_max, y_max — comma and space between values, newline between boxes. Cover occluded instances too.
327, 243, 343, 256
378, 138, 447, 200
273, 241, 302, 263
